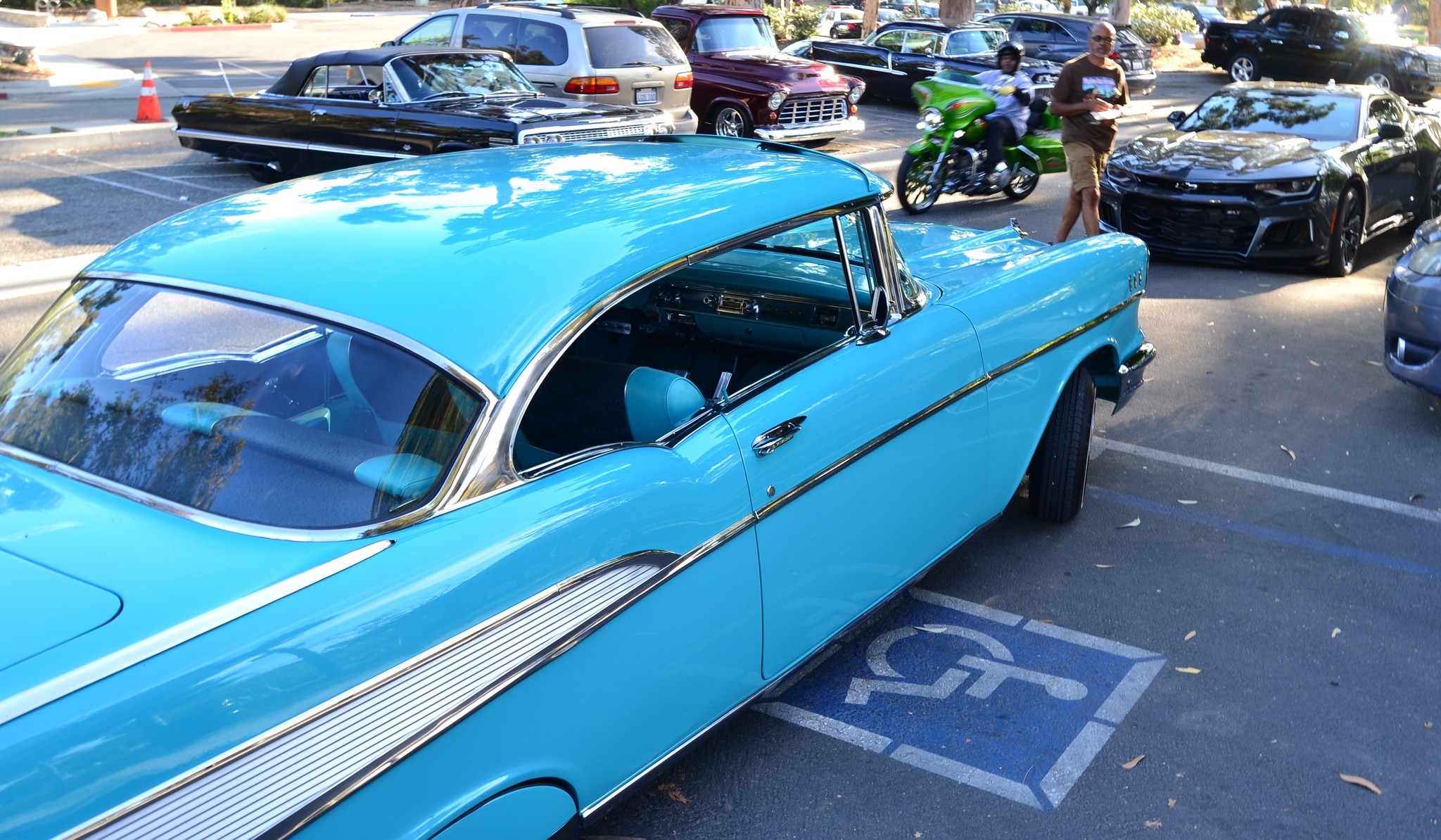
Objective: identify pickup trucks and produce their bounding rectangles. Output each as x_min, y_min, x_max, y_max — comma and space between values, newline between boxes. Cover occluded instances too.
651, 4, 866, 144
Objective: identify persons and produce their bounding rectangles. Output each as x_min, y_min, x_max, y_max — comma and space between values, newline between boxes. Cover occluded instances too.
937, 41, 1036, 185
1048, 21, 1131, 247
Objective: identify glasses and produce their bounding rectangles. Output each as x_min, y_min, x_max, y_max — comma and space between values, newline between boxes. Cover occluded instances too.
1091, 35, 1117, 43
999, 54, 1017, 62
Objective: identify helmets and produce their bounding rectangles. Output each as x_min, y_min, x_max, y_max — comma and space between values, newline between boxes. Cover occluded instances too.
996, 40, 1025, 64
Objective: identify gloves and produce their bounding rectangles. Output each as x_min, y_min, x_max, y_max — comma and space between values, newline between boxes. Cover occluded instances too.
999, 84, 1017, 96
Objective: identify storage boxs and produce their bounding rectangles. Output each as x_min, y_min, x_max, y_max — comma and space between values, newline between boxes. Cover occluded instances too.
1039, 102, 1062, 130
1024, 136, 1067, 173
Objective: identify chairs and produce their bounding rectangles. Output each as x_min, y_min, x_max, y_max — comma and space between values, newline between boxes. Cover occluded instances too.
326, 331, 532, 469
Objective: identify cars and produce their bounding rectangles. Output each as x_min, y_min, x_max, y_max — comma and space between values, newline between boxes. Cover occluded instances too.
782, 0, 1158, 117
1382, 213, 1441, 398
169, 47, 675, 184
0, 135, 1157, 840
1098, 80, 1441, 278
1171, 0, 1441, 104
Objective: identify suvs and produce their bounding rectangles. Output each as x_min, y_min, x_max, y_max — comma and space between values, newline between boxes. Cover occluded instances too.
381, 4, 700, 136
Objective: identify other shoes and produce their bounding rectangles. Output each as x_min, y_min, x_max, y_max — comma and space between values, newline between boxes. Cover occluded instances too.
975, 160, 1001, 172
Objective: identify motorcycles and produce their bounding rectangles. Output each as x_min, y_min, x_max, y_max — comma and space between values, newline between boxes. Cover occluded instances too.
897, 68, 1052, 216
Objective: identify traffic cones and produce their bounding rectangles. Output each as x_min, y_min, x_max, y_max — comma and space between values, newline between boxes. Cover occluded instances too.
131, 62, 169, 124
